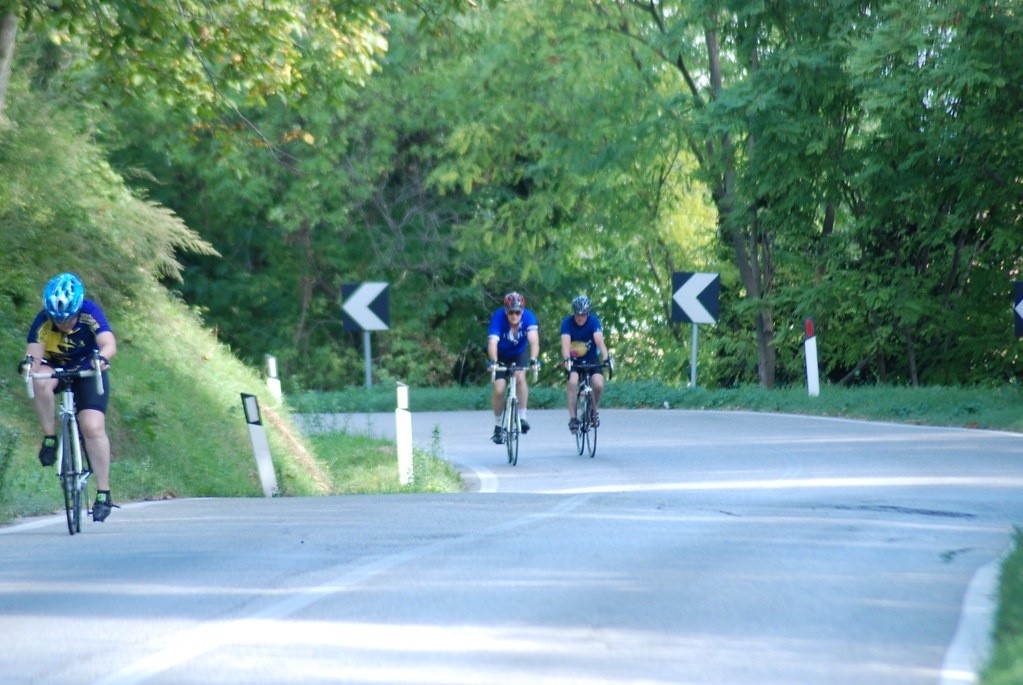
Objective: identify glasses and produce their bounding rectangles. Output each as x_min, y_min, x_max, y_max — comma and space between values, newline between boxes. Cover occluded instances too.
507, 310, 521, 315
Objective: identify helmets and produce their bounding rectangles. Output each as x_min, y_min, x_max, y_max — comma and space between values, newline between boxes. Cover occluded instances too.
572, 296, 592, 316
504, 292, 525, 312
43, 272, 84, 324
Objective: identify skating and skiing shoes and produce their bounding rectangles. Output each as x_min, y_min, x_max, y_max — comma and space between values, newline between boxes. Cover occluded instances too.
568, 418, 579, 430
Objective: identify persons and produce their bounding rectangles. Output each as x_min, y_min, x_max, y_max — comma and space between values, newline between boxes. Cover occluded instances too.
560, 296, 614, 432
486, 292, 542, 444
23, 271, 111, 520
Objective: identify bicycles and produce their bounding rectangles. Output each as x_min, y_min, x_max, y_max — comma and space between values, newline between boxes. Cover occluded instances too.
491, 359, 539, 467
16, 348, 110, 535
562, 355, 614, 459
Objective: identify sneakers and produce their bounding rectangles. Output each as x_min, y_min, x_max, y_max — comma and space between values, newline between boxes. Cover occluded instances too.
520, 419, 530, 430
38, 434, 58, 466
590, 409, 600, 427
490, 425, 503, 444
92, 490, 112, 521
